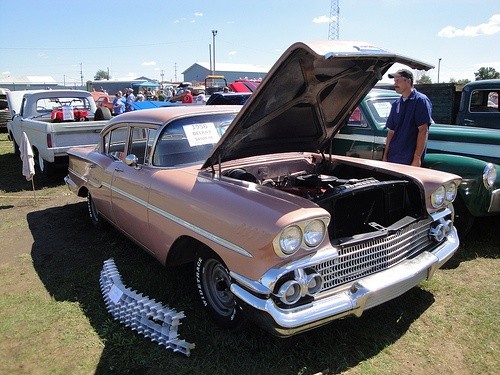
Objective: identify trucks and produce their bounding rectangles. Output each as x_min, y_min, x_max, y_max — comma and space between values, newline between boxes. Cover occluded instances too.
371, 78, 500, 130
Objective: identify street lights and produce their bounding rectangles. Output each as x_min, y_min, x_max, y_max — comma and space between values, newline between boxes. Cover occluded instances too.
437, 58, 443, 83
212, 29, 218, 83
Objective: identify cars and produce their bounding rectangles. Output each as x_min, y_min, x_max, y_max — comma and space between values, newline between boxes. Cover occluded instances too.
62, 39, 464, 338
324, 88, 500, 239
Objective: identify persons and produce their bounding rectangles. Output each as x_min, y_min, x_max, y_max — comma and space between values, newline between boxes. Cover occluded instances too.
47, 83, 236, 116
381, 69, 432, 168
486, 92, 499, 109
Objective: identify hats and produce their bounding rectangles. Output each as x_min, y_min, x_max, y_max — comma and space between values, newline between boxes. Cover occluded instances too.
388, 69, 414, 81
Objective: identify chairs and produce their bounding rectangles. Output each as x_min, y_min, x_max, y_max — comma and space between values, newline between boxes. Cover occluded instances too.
109, 141, 148, 165
162, 140, 212, 165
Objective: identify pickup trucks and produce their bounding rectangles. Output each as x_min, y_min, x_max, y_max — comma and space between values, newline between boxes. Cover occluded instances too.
7, 88, 115, 178
